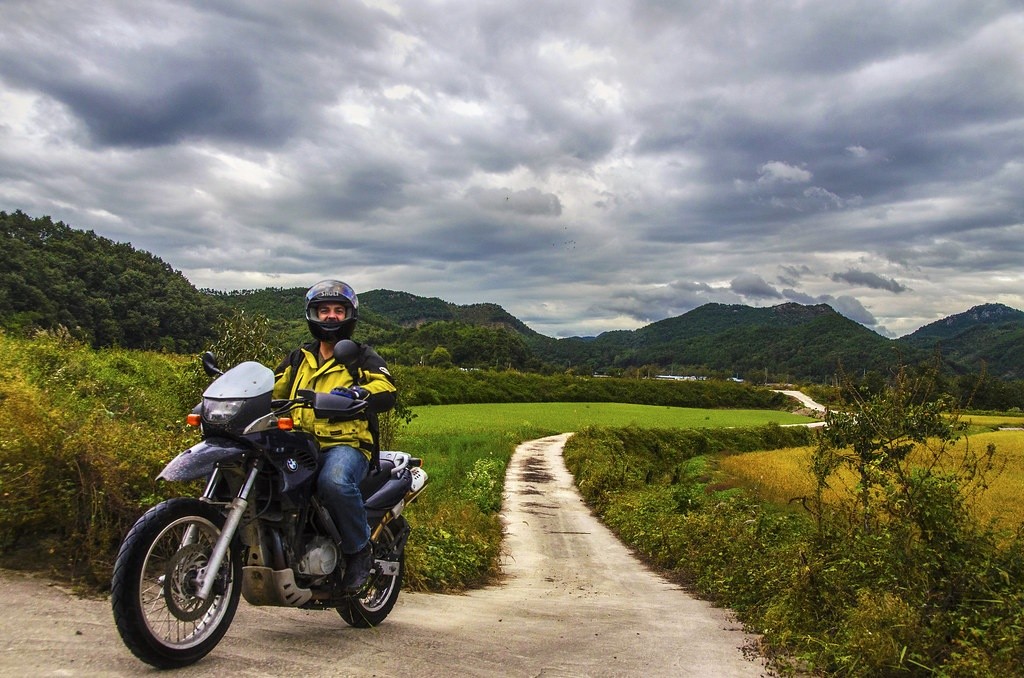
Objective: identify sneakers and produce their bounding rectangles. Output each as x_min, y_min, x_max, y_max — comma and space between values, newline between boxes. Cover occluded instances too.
340, 542, 373, 592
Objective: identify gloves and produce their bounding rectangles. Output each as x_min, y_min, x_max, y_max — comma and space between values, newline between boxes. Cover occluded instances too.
330, 385, 373, 399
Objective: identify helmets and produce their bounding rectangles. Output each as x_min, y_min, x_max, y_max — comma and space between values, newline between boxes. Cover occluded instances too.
305, 280, 359, 343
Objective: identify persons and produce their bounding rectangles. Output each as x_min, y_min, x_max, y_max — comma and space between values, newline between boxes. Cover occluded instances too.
272, 279, 398, 590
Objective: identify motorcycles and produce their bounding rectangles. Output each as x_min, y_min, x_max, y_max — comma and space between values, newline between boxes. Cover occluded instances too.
111, 338, 431, 670
